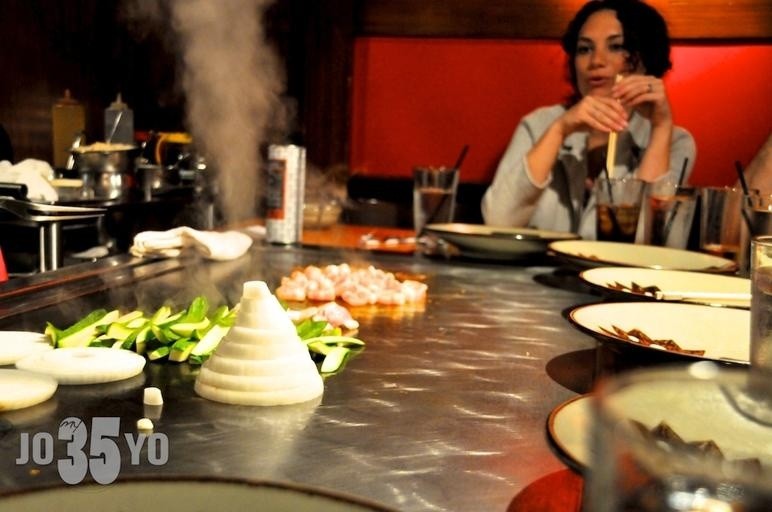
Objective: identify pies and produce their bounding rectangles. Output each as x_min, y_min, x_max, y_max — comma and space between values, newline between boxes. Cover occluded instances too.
0, 331, 146, 413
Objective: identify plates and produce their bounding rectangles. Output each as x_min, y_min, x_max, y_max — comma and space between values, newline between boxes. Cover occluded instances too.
560, 301, 751, 369
546, 239, 741, 276
578, 266, 752, 310
422, 221, 583, 260
545, 389, 593, 477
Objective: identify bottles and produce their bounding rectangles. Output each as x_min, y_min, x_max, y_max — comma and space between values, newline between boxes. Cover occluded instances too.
267, 143, 306, 246
51, 90, 87, 168
104, 94, 135, 146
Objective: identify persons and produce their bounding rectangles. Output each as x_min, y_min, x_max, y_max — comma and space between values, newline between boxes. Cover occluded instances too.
479, 1, 698, 249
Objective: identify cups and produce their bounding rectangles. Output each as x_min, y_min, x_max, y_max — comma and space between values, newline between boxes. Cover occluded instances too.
643, 181, 699, 250
700, 186, 772, 277
747, 234, 772, 406
595, 176, 646, 244
412, 166, 459, 249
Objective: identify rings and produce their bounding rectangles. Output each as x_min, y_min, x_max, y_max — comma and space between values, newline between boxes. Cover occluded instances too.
644, 81, 653, 91
